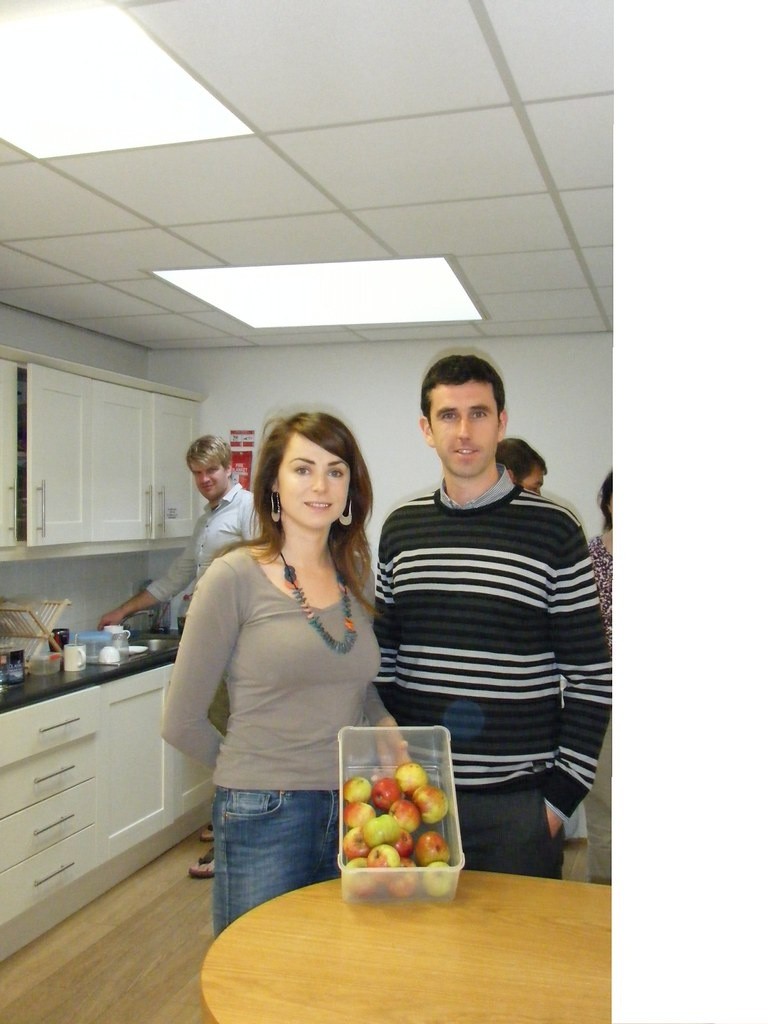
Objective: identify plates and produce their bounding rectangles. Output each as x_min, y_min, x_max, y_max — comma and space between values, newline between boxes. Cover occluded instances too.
119, 645, 149, 656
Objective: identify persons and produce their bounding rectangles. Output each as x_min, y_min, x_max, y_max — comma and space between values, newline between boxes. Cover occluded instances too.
160, 408, 411, 940
494, 437, 547, 501
100, 436, 292, 843
587, 468, 613, 654
372, 354, 610, 879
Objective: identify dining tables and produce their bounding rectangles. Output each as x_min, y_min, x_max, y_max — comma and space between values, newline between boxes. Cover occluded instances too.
200, 870, 611, 1024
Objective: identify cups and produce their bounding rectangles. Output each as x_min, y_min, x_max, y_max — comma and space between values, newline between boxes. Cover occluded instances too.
63, 644, 88, 673
54, 628, 70, 647
98, 646, 122, 665
50, 632, 60, 651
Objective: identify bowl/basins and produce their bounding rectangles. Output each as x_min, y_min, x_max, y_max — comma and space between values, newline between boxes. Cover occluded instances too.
133, 638, 182, 654
28, 650, 62, 676
71, 631, 112, 665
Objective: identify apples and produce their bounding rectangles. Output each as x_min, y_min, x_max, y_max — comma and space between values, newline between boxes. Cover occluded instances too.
344, 762, 452, 896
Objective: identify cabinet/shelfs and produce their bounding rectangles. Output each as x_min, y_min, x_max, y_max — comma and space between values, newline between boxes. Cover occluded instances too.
92, 367, 203, 556
0, 684, 101, 961
103, 663, 215, 860
0, 345, 92, 562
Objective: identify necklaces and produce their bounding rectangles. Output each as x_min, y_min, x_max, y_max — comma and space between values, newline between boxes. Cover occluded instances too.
269, 545, 359, 655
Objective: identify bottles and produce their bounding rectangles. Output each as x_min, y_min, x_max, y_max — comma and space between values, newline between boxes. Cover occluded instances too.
1, 644, 10, 695
178, 594, 192, 635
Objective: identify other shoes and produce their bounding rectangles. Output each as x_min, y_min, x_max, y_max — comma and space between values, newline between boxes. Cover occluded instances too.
189, 846, 214, 878
200, 824, 213, 841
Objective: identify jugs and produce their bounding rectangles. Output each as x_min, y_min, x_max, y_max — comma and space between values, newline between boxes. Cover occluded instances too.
102, 624, 132, 661
